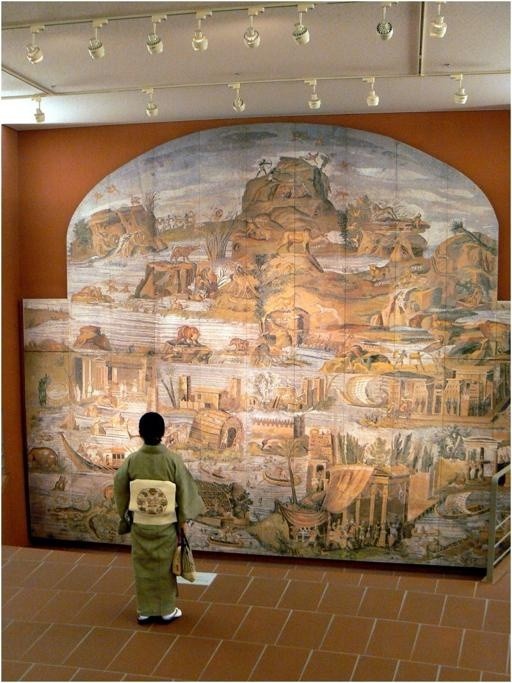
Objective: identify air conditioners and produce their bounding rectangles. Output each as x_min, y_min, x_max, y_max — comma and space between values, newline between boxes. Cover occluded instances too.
451, 73, 469, 107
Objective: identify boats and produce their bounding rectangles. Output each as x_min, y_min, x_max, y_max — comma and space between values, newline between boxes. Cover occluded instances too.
263, 469, 301, 488
59, 430, 131, 474
198, 462, 230, 481
206, 533, 243, 548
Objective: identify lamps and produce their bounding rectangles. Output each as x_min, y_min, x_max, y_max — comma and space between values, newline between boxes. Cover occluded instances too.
429, 2, 448, 38
25, 24, 48, 65
32, 96, 47, 124
376, 4, 395, 42
86, 20, 108, 61
192, 10, 214, 51
146, 15, 168, 54
142, 89, 158, 119
292, 4, 316, 47
243, 7, 265, 49
363, 78, 380, 106
304, 80, 323, 110
228, 82, 247, 113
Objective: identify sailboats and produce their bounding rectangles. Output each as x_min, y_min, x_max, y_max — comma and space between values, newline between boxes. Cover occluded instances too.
336, 374, 388, 407
434, 492, 490, 519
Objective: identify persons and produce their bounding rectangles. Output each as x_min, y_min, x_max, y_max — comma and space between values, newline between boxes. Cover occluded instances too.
113, 412, 207, 626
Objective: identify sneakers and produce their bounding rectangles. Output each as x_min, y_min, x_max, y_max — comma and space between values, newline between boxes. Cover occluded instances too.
137, 609, 180, 625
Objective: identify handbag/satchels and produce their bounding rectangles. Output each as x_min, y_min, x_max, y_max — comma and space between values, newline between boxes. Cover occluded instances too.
172, 527, 197, 583
